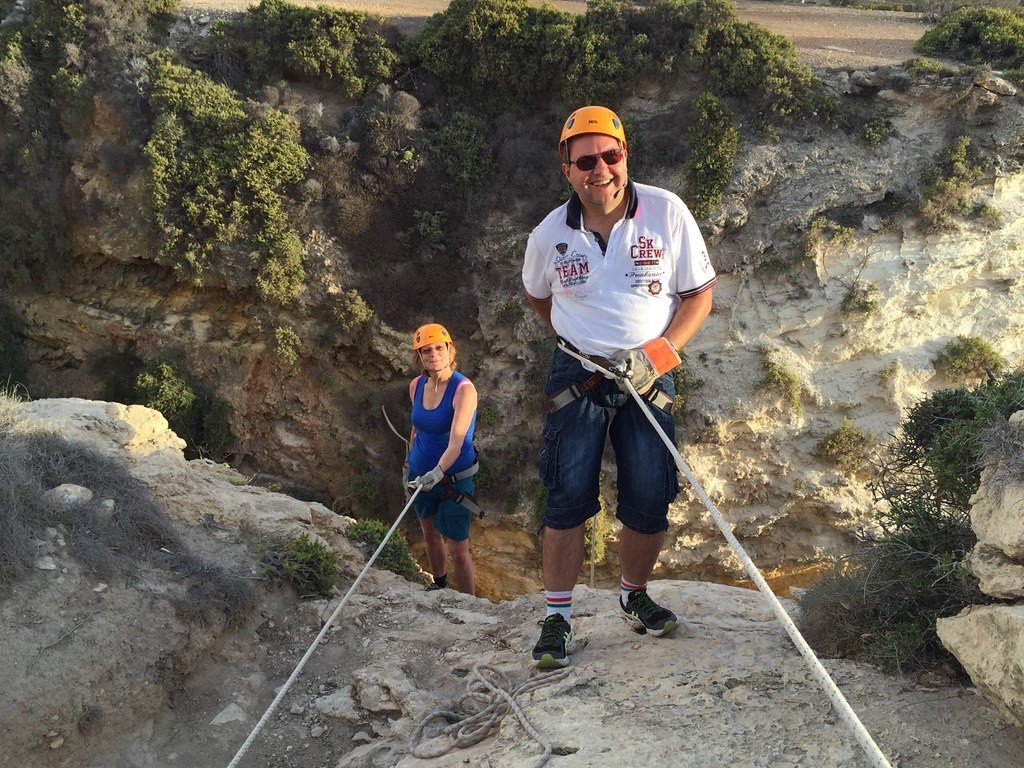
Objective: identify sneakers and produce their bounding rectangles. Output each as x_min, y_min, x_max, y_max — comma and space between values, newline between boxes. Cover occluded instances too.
532, 612, 573, 667
424, 582, 450, 591
619, 588, 678, 637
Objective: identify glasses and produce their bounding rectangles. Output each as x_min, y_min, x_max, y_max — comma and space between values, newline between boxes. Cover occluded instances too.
564, 149, 624, 172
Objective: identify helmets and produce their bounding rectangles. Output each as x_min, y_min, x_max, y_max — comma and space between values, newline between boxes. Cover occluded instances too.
413, 323, 453, 352
558, 106, 626, 160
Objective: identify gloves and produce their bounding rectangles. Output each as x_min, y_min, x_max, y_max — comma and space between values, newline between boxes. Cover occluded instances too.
403, 465, 411, 497
605, 336, 682, 396
414, 464, 445, 492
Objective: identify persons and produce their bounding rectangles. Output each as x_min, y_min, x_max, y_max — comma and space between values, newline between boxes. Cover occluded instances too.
403, 324, 480, 596
521, 105, 717, 668
